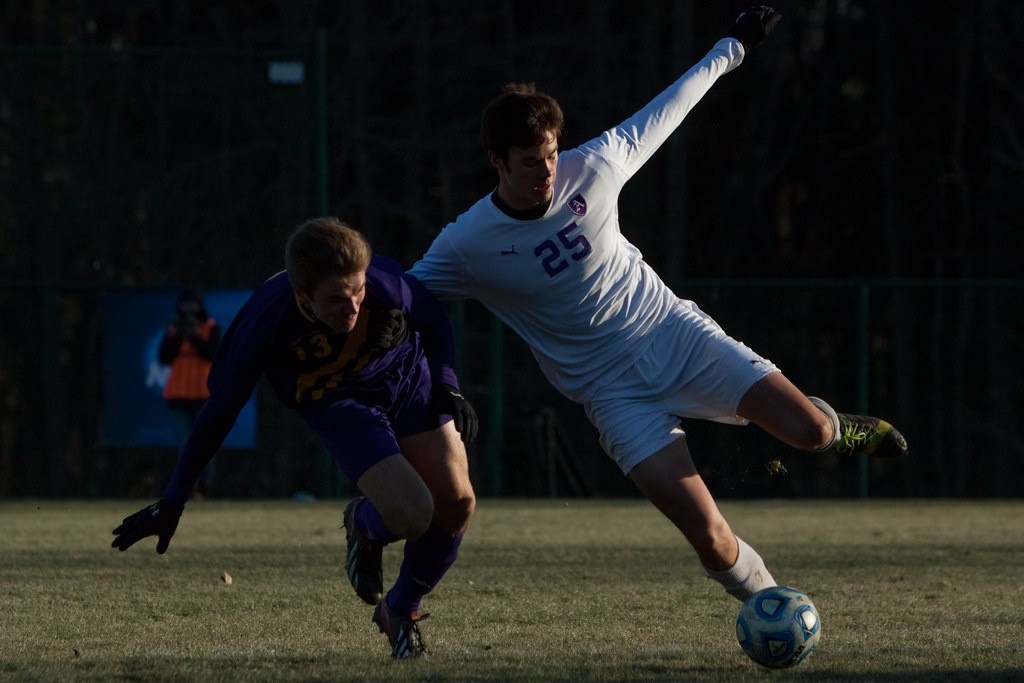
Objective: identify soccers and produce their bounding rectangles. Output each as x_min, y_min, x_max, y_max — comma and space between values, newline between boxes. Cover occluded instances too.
736, 586, 819, 668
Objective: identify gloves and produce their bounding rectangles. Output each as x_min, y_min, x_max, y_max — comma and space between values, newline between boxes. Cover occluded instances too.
365, 305, 410, 349
428, 383, 478, 443
726, 4, 783, 55
111, 499, 185, 555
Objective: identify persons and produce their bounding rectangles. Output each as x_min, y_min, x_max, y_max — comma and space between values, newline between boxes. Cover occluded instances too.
113, 218, 480, 659
401, 4, 908, 611
158, 289, 223, 496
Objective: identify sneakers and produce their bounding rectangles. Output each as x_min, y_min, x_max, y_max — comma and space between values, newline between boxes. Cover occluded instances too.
832, 413, 909, 460
341, 495, 383, 606
373, 598, 433, 659
